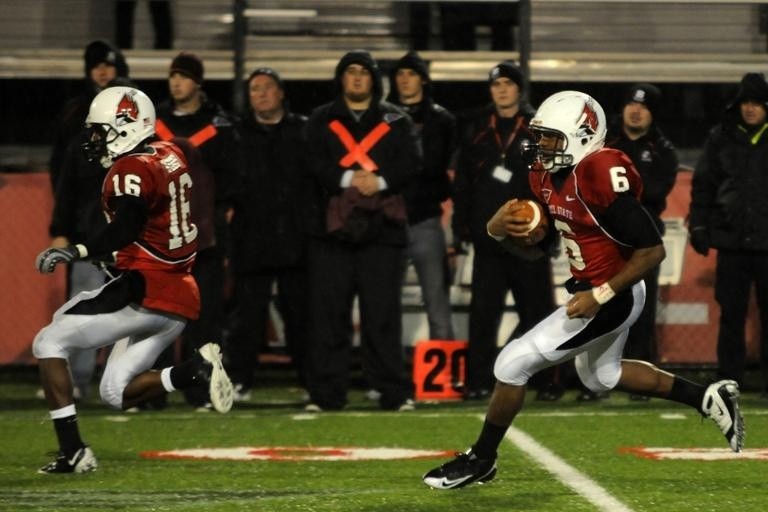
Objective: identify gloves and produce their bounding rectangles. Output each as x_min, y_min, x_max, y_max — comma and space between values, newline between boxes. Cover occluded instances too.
34, 243, 88, 275
690, 224, 712, 256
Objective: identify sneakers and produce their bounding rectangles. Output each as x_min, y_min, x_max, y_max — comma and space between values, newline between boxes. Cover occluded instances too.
462, 388, 490, 401
306, 401, 319, 411
701, 380, 745, 453
536, 382, 649, 402
36, 444, 98, 475
364, 387, 416, 413
192, 342, 233, 415
422, 448, 499, 490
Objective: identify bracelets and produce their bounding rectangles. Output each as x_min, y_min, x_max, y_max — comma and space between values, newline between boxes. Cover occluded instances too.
591, 282, 616, 307
483, 222, 507, 242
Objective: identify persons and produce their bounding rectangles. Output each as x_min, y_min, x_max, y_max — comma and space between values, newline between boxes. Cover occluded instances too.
687, 69, 768, 393
363, 48, 458, 400
304, 50, 424, 415
211, 64, 329, 403
451, 60, 559, 400
34, 40, 148, 403
31, 85, 233, 478
577, 78, 682, 404
147, 50, 235, 390
418, 91, 745, 492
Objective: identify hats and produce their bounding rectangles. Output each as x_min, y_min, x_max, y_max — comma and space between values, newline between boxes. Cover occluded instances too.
623, 83, 661, 117
169, 55, 203, 84
489, 61, 524, 90
249, 69, 281, 86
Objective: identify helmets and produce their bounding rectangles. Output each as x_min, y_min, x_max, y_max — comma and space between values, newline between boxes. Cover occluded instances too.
79, 86, 156, 169
84, 41, 128, 88
521, 91, 607, 174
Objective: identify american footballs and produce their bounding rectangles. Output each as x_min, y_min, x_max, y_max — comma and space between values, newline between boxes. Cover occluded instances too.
509, 199, 548, 247
